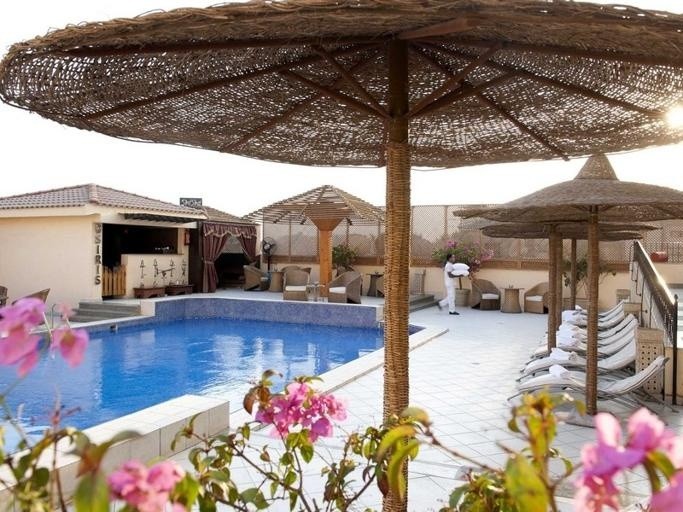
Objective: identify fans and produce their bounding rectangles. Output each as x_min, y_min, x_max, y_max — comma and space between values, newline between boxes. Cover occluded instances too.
261, 237, 278, 272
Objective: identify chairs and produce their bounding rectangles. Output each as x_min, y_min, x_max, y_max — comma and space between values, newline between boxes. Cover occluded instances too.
243, 265, 311, 301
471, 278, 549, 314
506, 299, 680, 423
326, 265, 364, 305
0, 286, 51, 328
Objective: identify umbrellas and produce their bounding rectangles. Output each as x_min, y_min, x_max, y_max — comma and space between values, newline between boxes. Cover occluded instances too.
2, 0, 683, 512
239, 184, 386, 297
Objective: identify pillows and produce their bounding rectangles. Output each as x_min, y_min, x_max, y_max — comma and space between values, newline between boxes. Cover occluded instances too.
451, 262, 470, 276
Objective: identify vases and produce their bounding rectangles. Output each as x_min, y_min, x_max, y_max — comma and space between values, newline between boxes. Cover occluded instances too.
454, 288, 470, 306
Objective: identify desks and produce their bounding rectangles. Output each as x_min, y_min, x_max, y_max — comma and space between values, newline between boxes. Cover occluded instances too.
366, 273, 385, 297
133, 284, 194, 298
308, 284, 325, 303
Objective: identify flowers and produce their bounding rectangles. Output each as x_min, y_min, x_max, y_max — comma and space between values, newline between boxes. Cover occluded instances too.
431, 239, 494, 288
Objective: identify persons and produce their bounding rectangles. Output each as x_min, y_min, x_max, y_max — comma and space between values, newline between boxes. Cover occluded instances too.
437, 253, 464, 315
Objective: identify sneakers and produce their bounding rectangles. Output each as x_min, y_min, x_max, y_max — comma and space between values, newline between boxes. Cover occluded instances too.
450, 312, 459, 314
